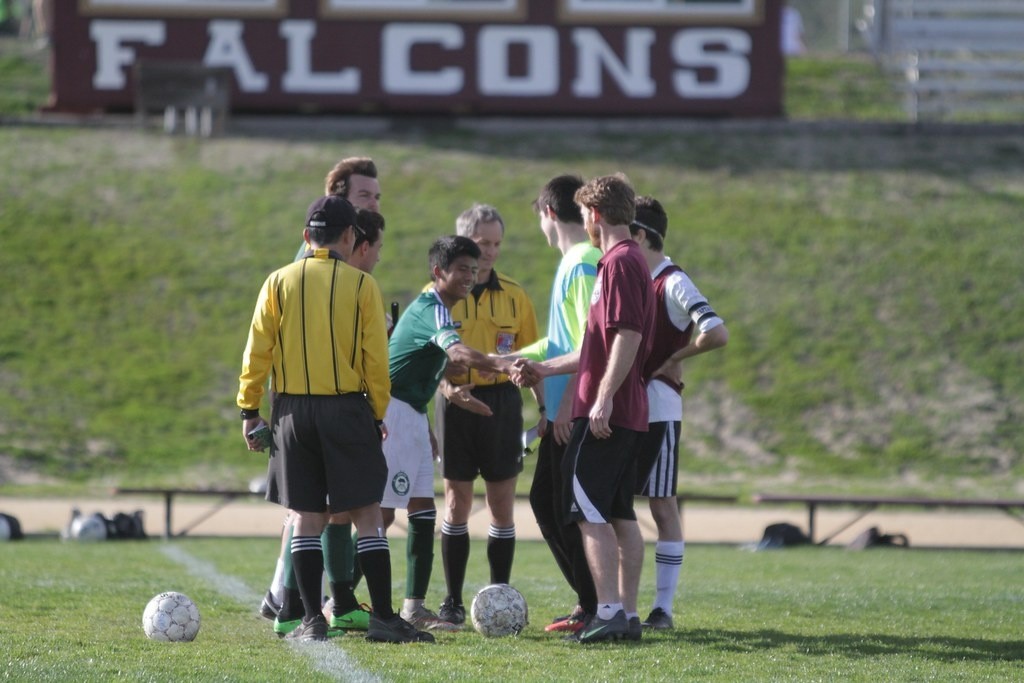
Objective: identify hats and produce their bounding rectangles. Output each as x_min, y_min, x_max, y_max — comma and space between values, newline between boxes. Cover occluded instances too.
305, 195, 366, 236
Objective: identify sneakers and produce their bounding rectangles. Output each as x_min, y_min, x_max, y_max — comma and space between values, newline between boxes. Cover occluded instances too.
622, 616, 642, 641
397, 605, 458, 631
639, 606, 673, 631
438, 595, 465, 625
329, 602, 372, 630
553, 615, 572, 624
259, 589, 281, 620
564, 609, 631, 645
273, 608, 344, 637
544, 605, 591, 632
322, 599, 334, 624
364, 607, 435, 644
283, 611, 327, 641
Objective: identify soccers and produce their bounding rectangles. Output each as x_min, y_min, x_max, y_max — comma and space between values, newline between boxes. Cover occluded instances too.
469, 582, 530, 639
70, 515, 107, 541
141, 591, 200, 644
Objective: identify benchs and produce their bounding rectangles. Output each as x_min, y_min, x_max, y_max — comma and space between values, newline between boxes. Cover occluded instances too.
114, 488, 739, 540
753, 494, 1024, 549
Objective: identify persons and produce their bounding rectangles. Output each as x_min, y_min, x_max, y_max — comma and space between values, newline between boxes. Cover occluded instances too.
237, 156, 729, 643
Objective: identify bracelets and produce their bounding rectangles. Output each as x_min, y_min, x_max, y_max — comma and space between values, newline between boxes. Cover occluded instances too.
240, 408, 260, 420
538, 406, 546, 412
377, 420, 384, 425
